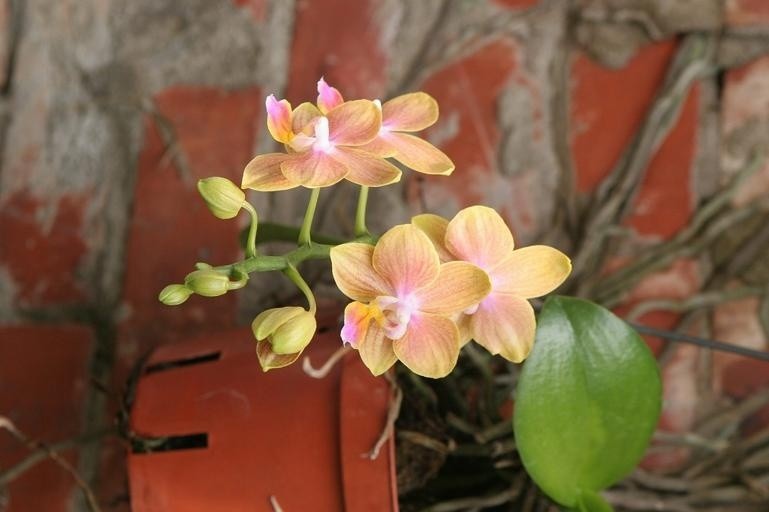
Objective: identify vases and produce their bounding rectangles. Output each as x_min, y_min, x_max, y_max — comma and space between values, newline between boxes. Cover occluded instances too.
125, 296, 400, 512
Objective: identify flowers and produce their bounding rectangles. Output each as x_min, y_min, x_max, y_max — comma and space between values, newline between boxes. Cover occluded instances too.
155, 33, 769, 508
